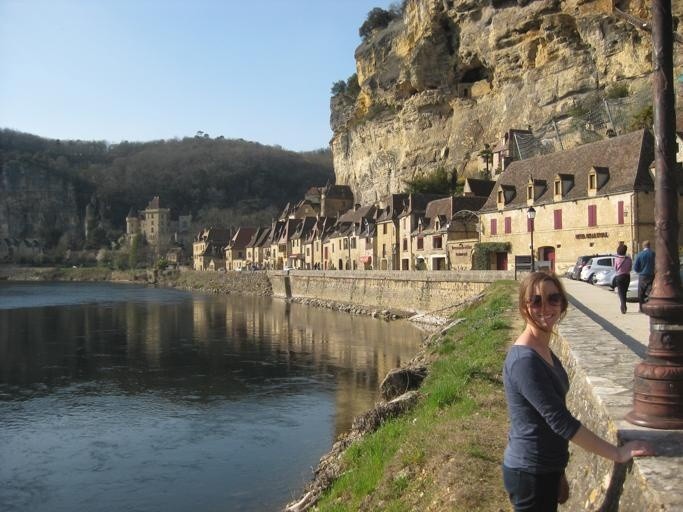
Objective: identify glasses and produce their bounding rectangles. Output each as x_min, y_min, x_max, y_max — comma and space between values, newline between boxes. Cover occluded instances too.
523, 294, 563, 309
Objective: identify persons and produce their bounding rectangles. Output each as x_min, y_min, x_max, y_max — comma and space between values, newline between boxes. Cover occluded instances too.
632, 239, 655, 313
613, 245, 632, 313
499, 270, 661, 511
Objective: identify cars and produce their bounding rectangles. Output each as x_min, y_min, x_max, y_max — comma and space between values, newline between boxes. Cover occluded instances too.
568, 253, 683, 305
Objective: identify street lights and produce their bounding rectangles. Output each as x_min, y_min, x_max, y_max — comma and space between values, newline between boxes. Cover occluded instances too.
525, 205, 537, 273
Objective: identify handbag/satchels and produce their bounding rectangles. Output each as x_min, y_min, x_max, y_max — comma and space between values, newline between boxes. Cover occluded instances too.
612, 276, 617, 288
